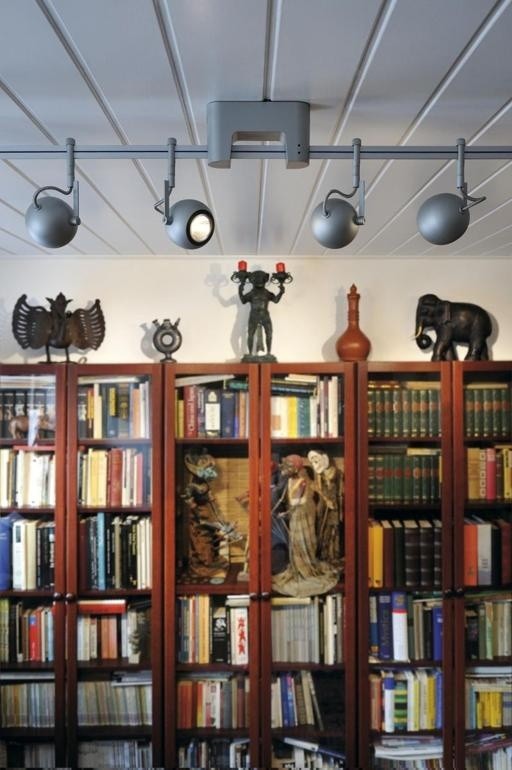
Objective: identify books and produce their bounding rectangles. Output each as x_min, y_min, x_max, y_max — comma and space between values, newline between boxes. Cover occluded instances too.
463, 381, 512, 770
174, 371, 249, 770
76, 374, 152, 767
271, 373, 347, 769
1, 373, 55, 769
367, 371, 444, 770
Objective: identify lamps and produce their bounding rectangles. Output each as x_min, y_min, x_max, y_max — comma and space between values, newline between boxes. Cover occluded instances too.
23, 136, 486, 249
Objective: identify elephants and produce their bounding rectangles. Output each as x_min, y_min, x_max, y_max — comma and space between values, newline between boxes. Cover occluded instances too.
414, 294, 493, 362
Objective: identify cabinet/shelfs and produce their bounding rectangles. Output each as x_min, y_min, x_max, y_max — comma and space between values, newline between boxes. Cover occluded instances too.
162, 360, 357, 769
0, 359, 164, 768
355, 359, 512, 770
